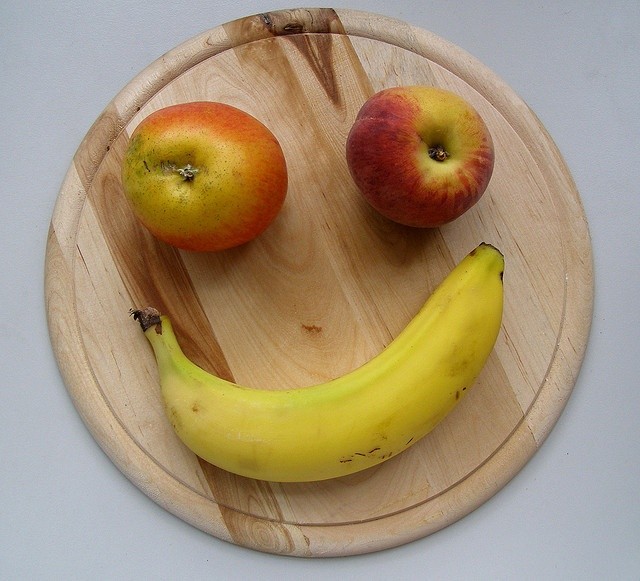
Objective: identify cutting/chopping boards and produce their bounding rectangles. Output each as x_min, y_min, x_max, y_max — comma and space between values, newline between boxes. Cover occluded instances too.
44, 6, 594, 558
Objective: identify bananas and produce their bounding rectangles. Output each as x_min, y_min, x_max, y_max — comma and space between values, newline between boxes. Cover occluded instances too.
130, 242, 505, 483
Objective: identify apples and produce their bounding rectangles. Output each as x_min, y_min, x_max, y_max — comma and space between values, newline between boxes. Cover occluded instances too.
122, 102, 290, 253
345, 85, 496, 227
